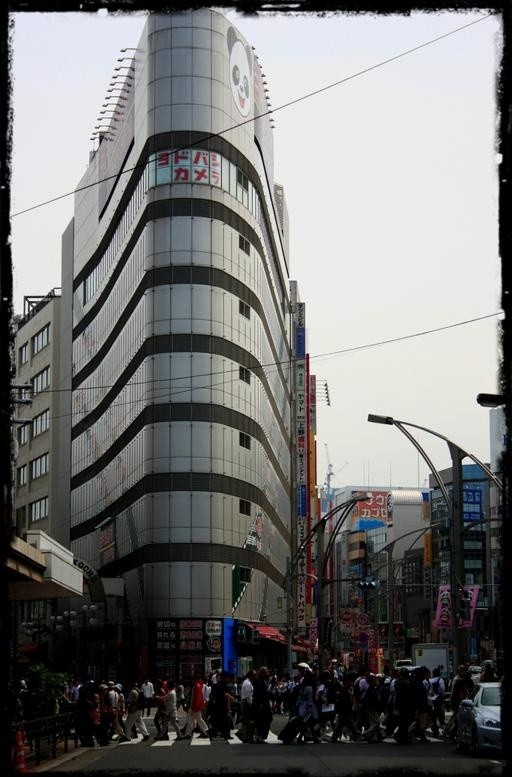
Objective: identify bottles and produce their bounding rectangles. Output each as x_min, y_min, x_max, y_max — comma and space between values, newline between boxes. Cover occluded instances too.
108, 681, 123, 691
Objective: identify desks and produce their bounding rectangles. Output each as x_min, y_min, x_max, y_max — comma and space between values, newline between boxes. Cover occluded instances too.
367, 394, 507, 657
282, 495, 371, 675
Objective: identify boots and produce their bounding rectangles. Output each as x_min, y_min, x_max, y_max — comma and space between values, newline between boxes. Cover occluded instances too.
395, 660, 413, 672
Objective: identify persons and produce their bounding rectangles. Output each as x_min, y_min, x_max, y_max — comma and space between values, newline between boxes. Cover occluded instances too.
1, 654, 511, 744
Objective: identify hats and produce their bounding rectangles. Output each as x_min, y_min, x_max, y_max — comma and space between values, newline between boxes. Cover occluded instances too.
468, 666, 482, 673
454, 682, 502, 754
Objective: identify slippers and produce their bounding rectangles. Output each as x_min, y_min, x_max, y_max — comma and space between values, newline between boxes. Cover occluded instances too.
432, 677, 443, 695
378, 677, 387, 691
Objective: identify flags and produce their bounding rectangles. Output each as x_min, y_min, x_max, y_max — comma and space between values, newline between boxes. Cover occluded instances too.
455, 585, 480, 628
433, 584, 453, 629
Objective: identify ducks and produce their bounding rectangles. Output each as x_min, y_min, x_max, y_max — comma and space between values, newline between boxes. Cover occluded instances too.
278, 711, 313, 746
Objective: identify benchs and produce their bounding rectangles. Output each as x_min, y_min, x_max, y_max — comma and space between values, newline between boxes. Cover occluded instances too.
433, 584, 453, 629
455, 585, 480, 628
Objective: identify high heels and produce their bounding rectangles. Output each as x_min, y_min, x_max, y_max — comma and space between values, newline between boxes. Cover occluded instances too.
14, 732, 28, 774
